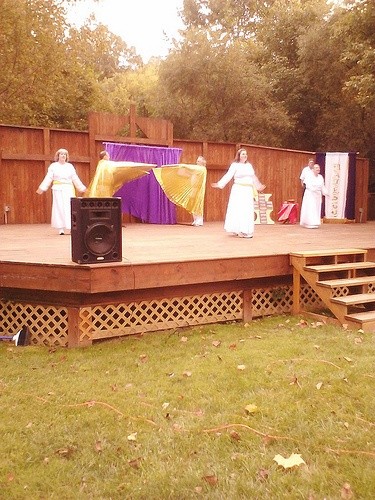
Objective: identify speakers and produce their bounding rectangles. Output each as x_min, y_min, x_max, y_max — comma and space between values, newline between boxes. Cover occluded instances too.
70, 197, 122, 264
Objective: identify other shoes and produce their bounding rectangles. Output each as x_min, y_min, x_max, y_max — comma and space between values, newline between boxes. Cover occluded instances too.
59, 227, 65, 235
235, 232, 246, 238
305, 224, 318, 229
243, 232, 253, 238
192, 217, 203, 226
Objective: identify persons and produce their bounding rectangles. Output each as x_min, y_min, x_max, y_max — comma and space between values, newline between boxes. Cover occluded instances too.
210, 149, 266, 238
152, 155, 206, 227
299, 164, 328, 229
82, 151, 157, 197
299, 159, 315, 187
35, 149, 89, 235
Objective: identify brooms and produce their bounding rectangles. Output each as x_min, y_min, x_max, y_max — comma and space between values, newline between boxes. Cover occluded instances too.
0, 324, 29, 347
